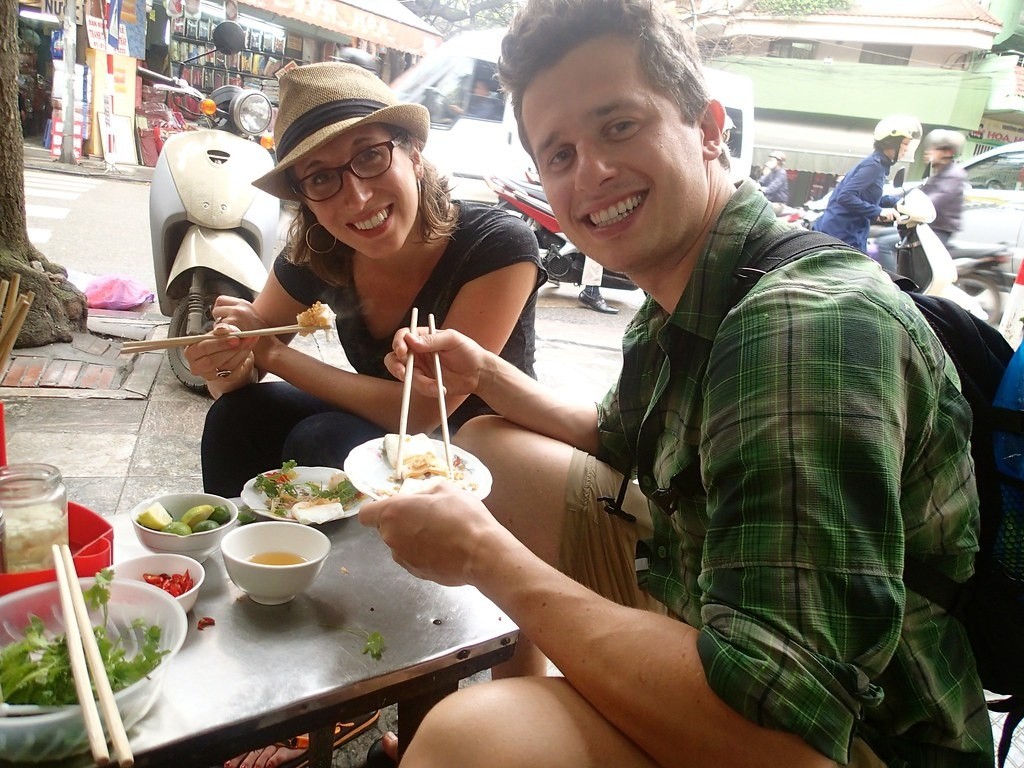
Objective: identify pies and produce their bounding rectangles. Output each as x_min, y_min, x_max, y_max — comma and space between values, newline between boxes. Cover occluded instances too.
292, 501, 345, 525
384, 432, 450, 482
399, 477, 452, 495
296, 303, 335, 341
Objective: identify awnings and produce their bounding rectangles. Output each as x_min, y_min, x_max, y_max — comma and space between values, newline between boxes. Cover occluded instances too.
750, 110, 936, 180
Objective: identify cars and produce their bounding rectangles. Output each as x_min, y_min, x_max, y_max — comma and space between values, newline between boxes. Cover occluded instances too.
881, 141, 1024, 257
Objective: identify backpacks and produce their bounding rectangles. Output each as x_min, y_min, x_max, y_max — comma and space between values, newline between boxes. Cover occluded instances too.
690, 230, 1024, 768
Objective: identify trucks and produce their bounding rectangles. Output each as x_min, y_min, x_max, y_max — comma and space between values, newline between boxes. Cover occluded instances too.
377, 24, 756, 205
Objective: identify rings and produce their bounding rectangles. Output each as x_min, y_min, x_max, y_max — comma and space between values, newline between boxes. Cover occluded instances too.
215, 368, 231, 379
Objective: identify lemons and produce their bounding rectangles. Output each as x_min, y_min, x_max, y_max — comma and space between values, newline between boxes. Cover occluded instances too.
180, 504, 215, 528
162, 505, 230, 536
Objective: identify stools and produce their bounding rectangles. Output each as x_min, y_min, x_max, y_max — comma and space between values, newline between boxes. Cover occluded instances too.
43, 119, 52, 149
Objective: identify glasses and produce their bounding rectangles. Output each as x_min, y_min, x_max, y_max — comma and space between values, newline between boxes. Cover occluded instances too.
651, 459, 702, 516
290, 133, 408, 202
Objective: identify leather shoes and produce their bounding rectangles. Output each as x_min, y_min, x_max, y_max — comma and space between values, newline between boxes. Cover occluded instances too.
578, 290, 619, 313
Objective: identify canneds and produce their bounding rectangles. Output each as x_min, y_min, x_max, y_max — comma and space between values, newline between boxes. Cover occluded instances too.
0, 462, 69, 573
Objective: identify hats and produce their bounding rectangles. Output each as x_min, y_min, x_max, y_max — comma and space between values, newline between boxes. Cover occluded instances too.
250, 62, 431, 201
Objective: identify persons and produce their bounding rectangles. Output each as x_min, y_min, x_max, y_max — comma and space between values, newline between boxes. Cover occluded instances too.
810, 112, 923, 255
182, 59, 549, 768
330, 46, 383, 81
879, 129, 989, 289
578, 256, 619, 316
439, 77, 499, 125
360, 0, 996, 768
756, 150, 789, 217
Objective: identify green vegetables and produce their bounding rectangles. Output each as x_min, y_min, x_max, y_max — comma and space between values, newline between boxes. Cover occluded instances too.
0, 569, 170, 762
316, 624, 384, 659
237, 460, 359, 523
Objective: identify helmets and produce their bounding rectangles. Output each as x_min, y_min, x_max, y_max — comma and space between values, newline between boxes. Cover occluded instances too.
925, 129, 965, 158
874, 115, 918, 147
769, 151, 786, 162
836, 176, 844, 182
723, 115, 736, 133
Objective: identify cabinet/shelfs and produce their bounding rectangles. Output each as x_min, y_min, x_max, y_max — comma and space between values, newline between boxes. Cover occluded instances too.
164, 2, 311, 109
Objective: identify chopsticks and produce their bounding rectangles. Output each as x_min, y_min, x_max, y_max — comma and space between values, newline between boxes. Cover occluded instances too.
120, 324, 332, 354
51, 542, 137, 767
392, 307, 457, 482
0, 273, 36, 376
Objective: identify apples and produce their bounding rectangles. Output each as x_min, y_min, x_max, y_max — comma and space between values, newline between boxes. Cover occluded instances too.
137, 502, 171, 529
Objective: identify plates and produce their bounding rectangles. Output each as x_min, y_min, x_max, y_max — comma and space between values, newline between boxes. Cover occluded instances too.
240, 466, 375, 525
344, 435, 494, 502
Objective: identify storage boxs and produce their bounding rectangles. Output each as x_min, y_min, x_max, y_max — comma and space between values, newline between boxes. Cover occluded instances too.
50, 59, 92, 159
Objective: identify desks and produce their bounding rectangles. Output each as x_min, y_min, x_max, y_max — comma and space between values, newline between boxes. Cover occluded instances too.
101, 500, 519, 768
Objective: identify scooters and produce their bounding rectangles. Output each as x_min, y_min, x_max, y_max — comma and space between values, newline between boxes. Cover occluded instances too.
484, 173, 640, 291
793, 160, 1012, 325
136, 19, 302, 397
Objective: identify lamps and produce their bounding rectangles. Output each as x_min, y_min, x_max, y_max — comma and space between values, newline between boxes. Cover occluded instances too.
19, 5, 60, 23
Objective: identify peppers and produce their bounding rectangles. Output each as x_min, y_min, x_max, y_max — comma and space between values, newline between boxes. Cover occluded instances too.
141, 569, 193, 600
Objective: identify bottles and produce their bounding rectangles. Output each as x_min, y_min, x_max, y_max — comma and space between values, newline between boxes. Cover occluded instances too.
0, 462, 69, 573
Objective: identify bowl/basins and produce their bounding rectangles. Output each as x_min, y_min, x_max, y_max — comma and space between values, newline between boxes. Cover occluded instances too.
129, 492, 239, 564
220, 521, 332, 606
0, 576, 189, 760
111, 553, 206, 613
